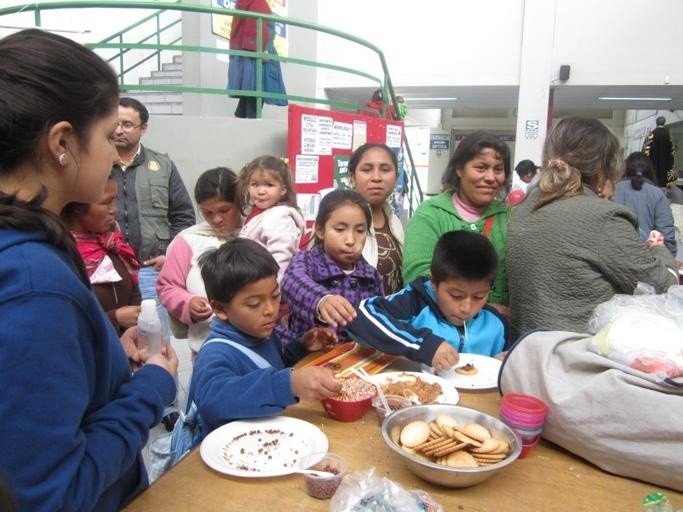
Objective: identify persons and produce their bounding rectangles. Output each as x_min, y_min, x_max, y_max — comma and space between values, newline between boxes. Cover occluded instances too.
107, 95, 196, 347
278, 187, 385, 350
235, 153, 307, 287
503, 114, 681, 352
227, 0, 290, 117
357, 88, 395, 121
0, 26, 181, 511
400, 130, 514, 307
182, 238, 344, 433
611, 150, 677, 260
516, 158, 544, 190
642, 115, 676, 187
153, 164, 247, 422
346, 230, 512, 375
58, 166, 144, 340
391, 93, 408, 122
346, 141, 404, 299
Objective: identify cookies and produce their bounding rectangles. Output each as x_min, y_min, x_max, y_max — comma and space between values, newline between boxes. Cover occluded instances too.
454, 365, 480, 375
394, 412, 513, 471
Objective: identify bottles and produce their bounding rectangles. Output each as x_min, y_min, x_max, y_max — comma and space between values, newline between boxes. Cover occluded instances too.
136, 300, 162, 367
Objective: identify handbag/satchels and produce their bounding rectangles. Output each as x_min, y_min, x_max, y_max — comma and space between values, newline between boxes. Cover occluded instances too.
145, 416, 202, 486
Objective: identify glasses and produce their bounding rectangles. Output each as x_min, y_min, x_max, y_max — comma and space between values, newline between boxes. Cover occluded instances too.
116, 121, 144, 134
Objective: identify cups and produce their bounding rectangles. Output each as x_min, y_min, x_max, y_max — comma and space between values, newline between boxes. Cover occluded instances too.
500, 393, 547, 458
300, 452, 346, 499
371, 394, 414, 425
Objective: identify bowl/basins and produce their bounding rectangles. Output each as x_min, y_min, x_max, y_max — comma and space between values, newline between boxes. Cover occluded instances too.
382, 403, 523, 488
320, 376, 376, 421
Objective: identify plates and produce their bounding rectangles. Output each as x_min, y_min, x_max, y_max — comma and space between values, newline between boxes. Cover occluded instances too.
419, 352, 502, 390
199, 415, 329, 478
360, 371, 459, 405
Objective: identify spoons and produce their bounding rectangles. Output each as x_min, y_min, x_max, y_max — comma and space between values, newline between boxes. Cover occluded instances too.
300, 469, 334, 479
